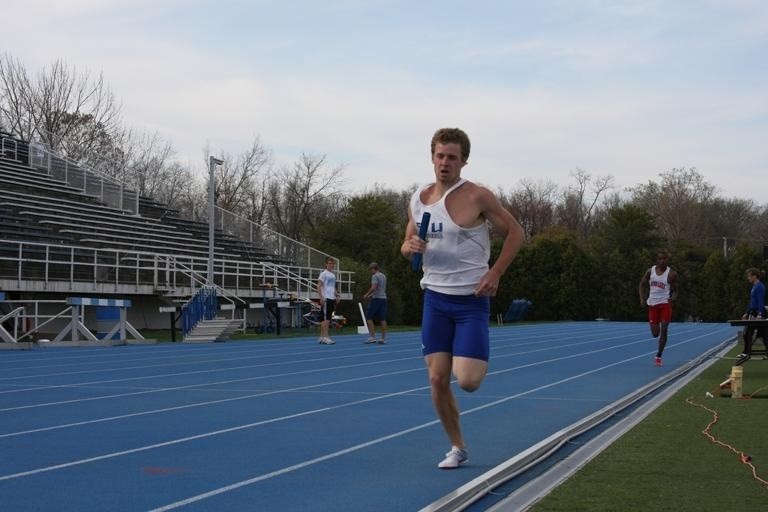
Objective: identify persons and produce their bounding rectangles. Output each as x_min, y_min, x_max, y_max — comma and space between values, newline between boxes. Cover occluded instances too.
363, 262, 388, 344
317, 257, 341, 345
638, 250, 680, 367
400, 128, 525, 469
736, 268, 768, 357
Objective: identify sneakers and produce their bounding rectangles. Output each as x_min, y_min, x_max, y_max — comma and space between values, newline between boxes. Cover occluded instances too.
655, 355, 664, 368
319, 337, 336, 345
437, 445, 469, 469
736, 353, 748, 358
365, 337, 387, 344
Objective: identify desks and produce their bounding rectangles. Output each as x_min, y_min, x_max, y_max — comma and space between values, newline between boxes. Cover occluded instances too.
729, 317, 768, 368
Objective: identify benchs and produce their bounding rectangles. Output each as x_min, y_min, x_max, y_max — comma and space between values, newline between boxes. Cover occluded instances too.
1, 133, 327, 291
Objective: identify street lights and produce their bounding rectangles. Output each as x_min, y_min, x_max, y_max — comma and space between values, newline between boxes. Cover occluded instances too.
208, 155, 225, 287
